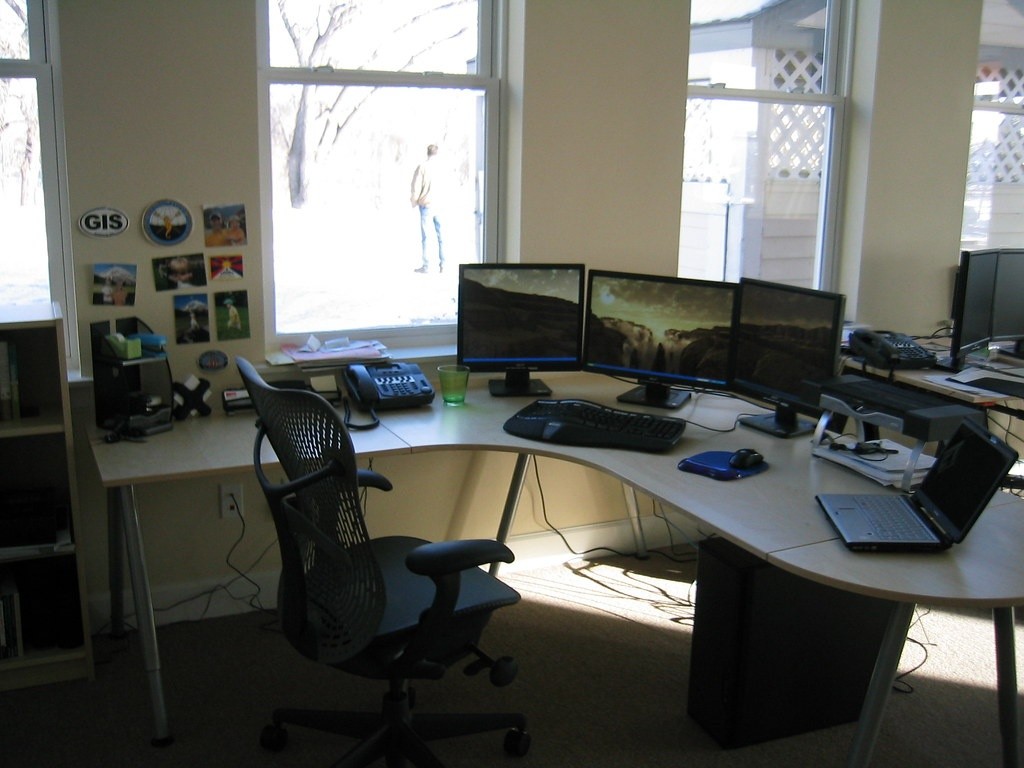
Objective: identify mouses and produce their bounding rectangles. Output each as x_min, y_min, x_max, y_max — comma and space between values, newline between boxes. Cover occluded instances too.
729, 448, 763, 470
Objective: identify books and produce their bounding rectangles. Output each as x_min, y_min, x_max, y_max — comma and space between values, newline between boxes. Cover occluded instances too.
0, 582, 23, 658
0, 341, 20, 421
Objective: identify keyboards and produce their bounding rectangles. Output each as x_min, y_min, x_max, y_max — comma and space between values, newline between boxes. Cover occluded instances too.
503, 398, 686, 452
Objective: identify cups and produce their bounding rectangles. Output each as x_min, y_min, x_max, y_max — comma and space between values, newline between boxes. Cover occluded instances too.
437, 365, 470, 402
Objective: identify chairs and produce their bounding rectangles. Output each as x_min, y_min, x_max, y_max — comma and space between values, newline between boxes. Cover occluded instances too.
233, 354, 532, 768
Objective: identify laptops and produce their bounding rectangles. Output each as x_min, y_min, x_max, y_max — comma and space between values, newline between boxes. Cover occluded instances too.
815, 414, 1019, 552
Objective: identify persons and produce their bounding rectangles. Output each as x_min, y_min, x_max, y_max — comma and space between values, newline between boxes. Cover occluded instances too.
102, 211, 244, 332
413, 144, 446, 271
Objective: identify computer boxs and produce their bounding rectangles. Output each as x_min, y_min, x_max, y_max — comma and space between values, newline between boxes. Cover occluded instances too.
688, 535, 899, 750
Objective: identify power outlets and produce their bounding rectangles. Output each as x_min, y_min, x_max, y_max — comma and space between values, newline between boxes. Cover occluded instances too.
218, 482, 245, 519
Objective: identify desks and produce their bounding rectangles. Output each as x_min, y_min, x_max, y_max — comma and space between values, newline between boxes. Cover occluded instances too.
766, 501, 1024, 768
841, 338, 1024, 423
83, 405, 1021, 768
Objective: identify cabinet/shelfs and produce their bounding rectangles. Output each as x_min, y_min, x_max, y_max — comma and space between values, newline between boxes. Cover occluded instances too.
0, 319, 96, 693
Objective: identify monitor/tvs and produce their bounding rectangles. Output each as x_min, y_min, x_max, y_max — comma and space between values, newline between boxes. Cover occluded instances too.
457, 264, 586, 397
934, 249, 1000, 374
582, 269, 741, 410
730, 277, 847, 438
992, 248, 1024, 360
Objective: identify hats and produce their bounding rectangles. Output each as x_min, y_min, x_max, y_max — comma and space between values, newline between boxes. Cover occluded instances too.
228, 215, 241, 224
210, 212, 223, 220
223, 299, 232, 305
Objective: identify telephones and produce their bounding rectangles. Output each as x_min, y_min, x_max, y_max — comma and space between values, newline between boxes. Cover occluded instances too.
851, 330, 937, 373
344, 362, 435, 412
